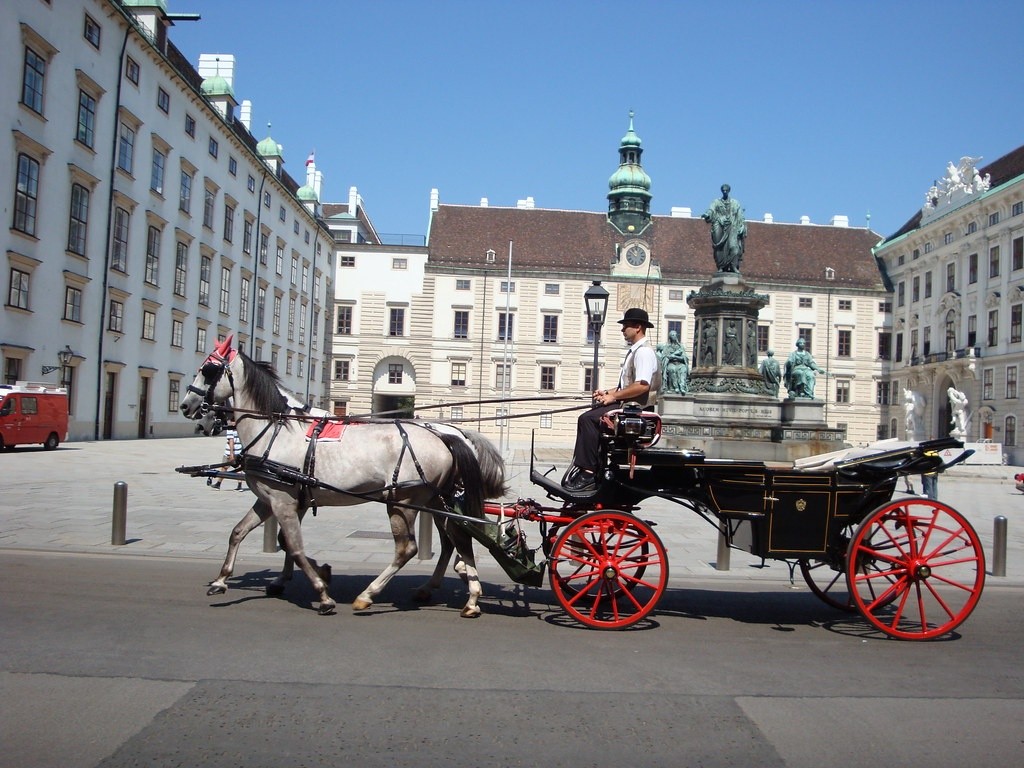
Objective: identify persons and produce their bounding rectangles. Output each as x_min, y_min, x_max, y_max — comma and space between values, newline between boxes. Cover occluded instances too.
212, 422, 243, 492
946, 386, 969, 432
903, 449, 940, 500
722, 320, 742, 365
903, 396, 916, 433
747, 321, 756, 366
759, 350, 781, 398
701, 184, 747, 272
783, 337, 825, 398
702, 320, 717, 367
564, 308, 661, 492
1014, 473, 1024, 494
657, 331, 690, 394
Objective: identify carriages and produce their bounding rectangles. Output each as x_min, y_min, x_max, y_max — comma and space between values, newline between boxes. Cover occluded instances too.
180, 331, 987, 641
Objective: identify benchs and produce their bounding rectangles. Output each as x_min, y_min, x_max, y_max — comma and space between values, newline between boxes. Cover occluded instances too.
598, 406, 662, 469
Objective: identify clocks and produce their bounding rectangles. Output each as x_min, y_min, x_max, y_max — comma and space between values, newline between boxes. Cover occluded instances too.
625, 246, 647, 267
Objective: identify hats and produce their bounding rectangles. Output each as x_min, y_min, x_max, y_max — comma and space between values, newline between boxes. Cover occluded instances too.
617, 308, 654, 328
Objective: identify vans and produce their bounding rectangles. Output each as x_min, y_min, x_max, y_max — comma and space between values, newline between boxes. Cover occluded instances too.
0, 382, 70, 452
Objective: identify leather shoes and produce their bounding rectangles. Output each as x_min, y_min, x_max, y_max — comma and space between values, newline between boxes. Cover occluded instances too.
565, 473, 582, 489
567, 475, 596, 491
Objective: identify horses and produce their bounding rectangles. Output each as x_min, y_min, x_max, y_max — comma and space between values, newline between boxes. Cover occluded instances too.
178, 333, 510, 619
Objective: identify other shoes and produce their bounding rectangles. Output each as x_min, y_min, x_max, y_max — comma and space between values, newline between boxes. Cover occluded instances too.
233, 488, 244, 492
210, 484, 220, 490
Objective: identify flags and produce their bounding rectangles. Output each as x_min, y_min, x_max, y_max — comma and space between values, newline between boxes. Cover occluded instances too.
305, 151, 315, 166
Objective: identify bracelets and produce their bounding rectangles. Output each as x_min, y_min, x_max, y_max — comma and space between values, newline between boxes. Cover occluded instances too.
603, 390, 609, 395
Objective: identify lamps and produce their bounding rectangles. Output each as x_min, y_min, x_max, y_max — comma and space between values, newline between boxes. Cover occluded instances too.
42, 345, 74, 376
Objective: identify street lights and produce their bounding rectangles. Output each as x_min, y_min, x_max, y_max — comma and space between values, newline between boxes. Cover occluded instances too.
581, 280, 610, 409
